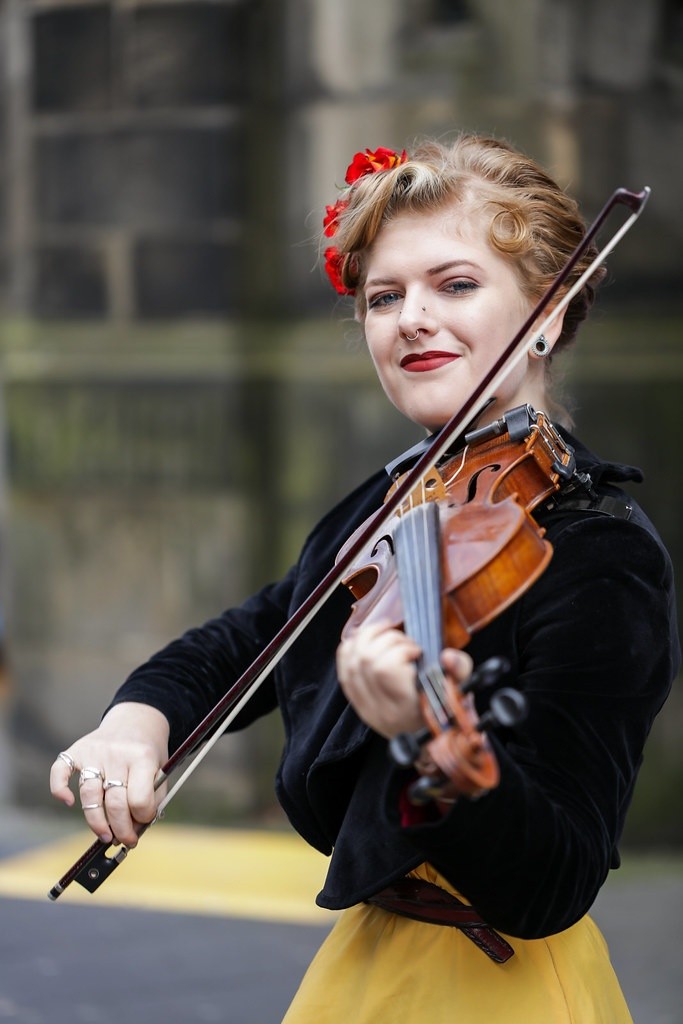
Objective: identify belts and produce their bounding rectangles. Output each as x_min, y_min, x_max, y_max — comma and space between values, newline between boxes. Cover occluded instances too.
364, 877, 515, 963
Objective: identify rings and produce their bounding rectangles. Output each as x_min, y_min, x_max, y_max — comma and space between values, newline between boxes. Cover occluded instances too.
81, 804, 102, 809
78, 767, 104, 786
58, 751, 74, 773
102, 780, 127, 789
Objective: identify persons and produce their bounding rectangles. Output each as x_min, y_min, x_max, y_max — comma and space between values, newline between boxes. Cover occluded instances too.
50, 130, 683, 1023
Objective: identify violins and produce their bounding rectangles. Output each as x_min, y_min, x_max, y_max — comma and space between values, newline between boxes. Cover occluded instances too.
333, 404, 573, 819
